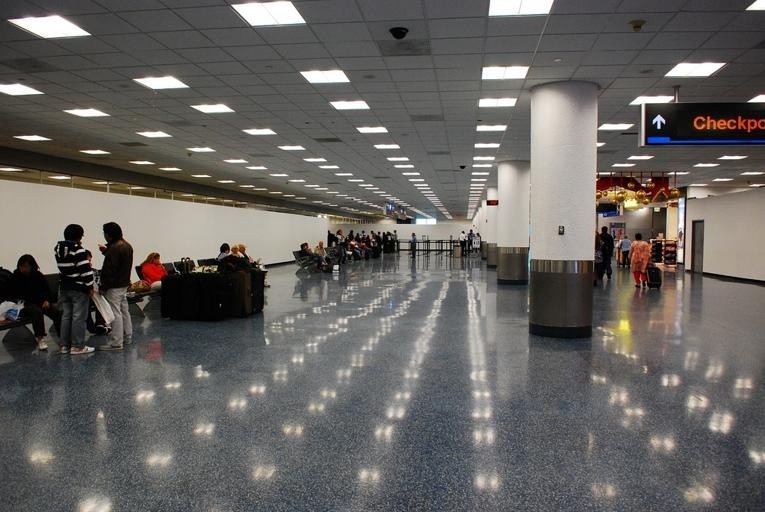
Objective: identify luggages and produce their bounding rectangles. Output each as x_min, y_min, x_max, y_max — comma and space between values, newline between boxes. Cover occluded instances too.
645, 264, 662, 289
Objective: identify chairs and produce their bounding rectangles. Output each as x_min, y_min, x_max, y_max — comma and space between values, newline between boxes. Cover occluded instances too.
135, 256, 267, 302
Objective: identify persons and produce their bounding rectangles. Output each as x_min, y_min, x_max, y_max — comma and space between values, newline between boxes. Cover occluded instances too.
300, 229, 398, 268
238, 243, 271, 287
460, 231, 467, 256
627, 232, 651, 289
231, 245, 241, 259
619, 236, 631, 269
140, 252, 168, 291
217, 243, 237, 271
594, 232, 606, 286
468, 230, 475, 252
600, 226, 614, 279
409, 232, 417, 258
1, 222, 133, 356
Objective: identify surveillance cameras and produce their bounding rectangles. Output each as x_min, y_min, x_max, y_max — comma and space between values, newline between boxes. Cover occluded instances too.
391, 26, 409, 39
627, 18, 648, 33
459, 166, 466, 169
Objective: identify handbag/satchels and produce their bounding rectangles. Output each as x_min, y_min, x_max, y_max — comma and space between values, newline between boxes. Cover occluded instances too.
127, 280, 151, 293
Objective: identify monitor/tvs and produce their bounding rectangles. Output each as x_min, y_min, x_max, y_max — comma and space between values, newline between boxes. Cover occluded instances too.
603, 206, 619, 217
597, 202, 618, 213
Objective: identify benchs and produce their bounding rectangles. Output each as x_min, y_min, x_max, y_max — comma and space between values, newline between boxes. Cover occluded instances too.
292, 248, 324, 274
0, 268, 106, 346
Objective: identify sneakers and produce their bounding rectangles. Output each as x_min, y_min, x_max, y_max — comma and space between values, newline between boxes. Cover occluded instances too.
60, 345, 96, 355
98, 340, 132, 351
96, 324, 112, 335
37, 339, 48, 351
